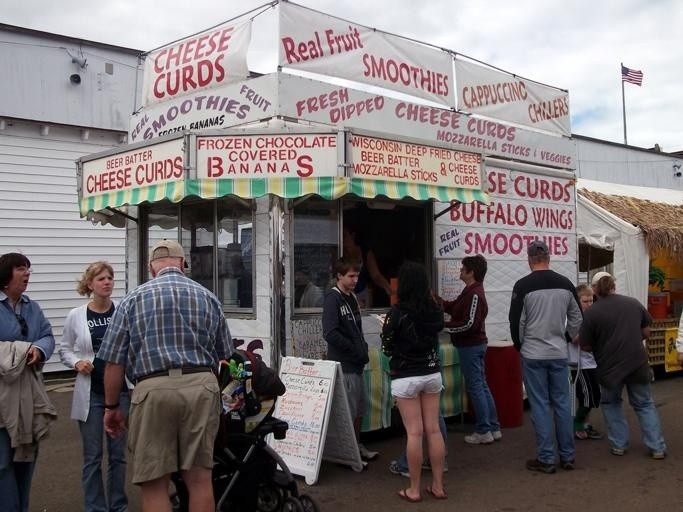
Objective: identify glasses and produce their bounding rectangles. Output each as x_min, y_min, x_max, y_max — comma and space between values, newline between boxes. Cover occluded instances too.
15, 314, 28, 336
17, 267, 32, 272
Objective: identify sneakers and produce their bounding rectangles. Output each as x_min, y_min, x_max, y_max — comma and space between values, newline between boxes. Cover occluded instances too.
526, 459, 555, 474
357, 443, 379, 460
584, 425, 603, 440
651, 452, 664, 459
463, 431, 493, 444
388, 460, 409, 477
490, 431, 501, 440
611, 448, 623, 456
560, 459, 574, 470
420, 457, 448, 472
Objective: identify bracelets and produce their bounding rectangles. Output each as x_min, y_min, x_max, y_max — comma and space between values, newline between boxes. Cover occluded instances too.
105, 402, 121, 410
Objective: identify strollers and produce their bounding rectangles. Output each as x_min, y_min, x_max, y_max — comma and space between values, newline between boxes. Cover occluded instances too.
168, 337, 322, 511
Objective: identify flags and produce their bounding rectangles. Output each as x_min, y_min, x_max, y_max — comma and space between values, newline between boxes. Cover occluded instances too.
622, 67, 642, 87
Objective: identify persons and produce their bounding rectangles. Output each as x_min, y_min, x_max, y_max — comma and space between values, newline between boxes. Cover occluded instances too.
57, 261, 134, 511
431, 254, 503, 446
380, 263, 449, 502
507, 239, 584, 475
388, 348, 450, 478
294, 266, 324, 310
320, 254, 380, 469
95, 240, 237, 511
344, 229, 392, 309
575, 270, 668, 460
1, 252, 57, 511
229, 254, 252, 308
674, 310, 683, 366
571, 285, 606, 440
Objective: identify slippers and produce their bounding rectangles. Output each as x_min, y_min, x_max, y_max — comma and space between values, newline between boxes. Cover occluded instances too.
398, 489, 422, 503
423, 484, 446, 499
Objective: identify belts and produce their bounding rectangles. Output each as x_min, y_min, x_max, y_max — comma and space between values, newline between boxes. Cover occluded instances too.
137, 366, 211, 383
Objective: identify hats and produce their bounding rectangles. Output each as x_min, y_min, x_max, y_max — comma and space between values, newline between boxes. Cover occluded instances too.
591, 271, 611, 285
149, 239, 188, 268
527, 241, 548, 256
